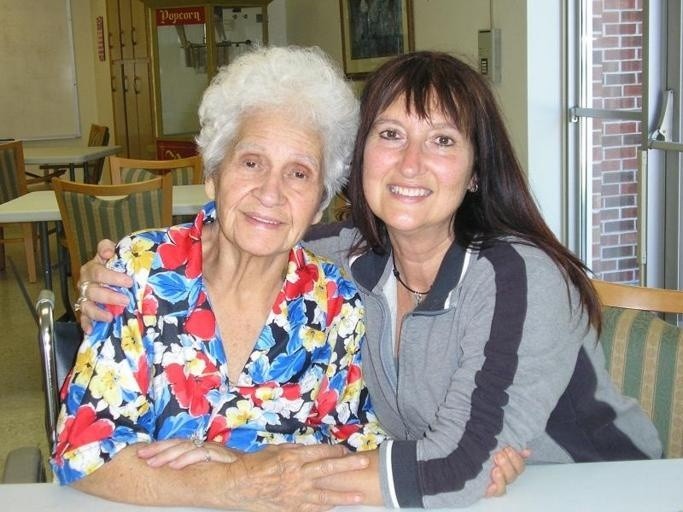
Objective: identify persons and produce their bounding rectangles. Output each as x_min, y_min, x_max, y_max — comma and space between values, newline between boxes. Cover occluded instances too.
46, 43, 531, 512
71, 49, 667, 510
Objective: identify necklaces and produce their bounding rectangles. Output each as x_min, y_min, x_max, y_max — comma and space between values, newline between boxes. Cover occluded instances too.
391, 245, 432, 307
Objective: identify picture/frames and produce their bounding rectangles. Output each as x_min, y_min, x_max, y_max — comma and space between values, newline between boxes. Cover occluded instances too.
336, 0, 414, 80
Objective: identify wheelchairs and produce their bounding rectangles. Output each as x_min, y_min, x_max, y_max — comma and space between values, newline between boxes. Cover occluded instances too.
0, 289, 83, 485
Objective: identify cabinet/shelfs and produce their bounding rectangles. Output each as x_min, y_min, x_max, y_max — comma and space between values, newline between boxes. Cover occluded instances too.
104, 0, 269, 162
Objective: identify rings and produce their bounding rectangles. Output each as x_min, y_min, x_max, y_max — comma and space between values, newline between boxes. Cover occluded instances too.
72, 295, 88, 314
79, 281, 92, 298
